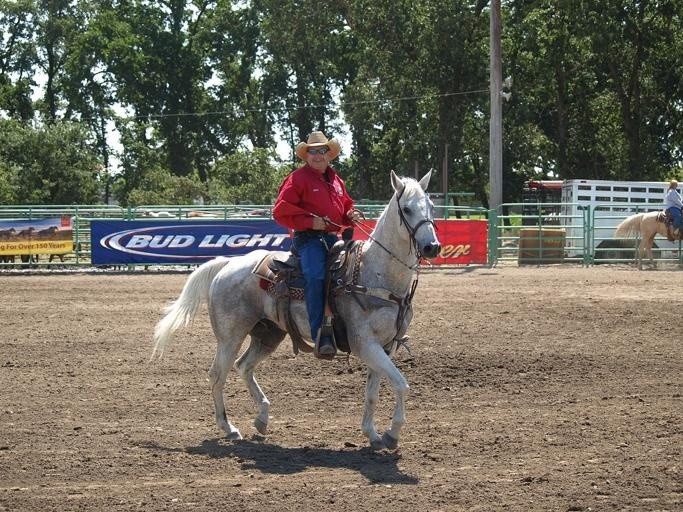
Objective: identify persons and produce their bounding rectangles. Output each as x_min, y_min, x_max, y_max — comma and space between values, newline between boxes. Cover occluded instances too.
272, 129, 356, 355
665, 180, 683, 234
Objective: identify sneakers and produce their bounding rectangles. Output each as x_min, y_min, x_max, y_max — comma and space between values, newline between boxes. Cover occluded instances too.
318, 343, 336, 355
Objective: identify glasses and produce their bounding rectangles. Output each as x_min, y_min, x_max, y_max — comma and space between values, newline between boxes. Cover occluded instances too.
306, 146, 329, 156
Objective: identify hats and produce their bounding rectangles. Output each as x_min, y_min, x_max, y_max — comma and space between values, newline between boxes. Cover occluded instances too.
295, 130, 342, 162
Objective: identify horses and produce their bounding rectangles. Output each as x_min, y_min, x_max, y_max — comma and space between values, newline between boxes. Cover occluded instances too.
148, 167, 442, 451
614, 210, 683, 271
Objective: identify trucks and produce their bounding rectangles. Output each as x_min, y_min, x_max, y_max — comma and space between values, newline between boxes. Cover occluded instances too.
522, 179, 682, 265
